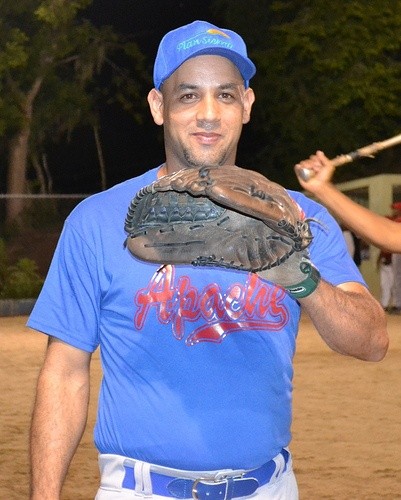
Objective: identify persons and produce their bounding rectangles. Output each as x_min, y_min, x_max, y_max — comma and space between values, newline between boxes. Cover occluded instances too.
29, 21, 389, 500
296, 151, 401, 253
343, 227, 361, 268
371, 216, 399, 311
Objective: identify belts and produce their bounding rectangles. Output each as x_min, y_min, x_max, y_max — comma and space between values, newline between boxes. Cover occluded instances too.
122, 447, 290, 500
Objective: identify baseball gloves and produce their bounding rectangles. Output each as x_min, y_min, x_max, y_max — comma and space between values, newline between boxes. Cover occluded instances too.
123, 163, 320, 303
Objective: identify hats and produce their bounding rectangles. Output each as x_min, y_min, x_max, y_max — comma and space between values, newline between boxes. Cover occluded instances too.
153, 19, 256, 91
